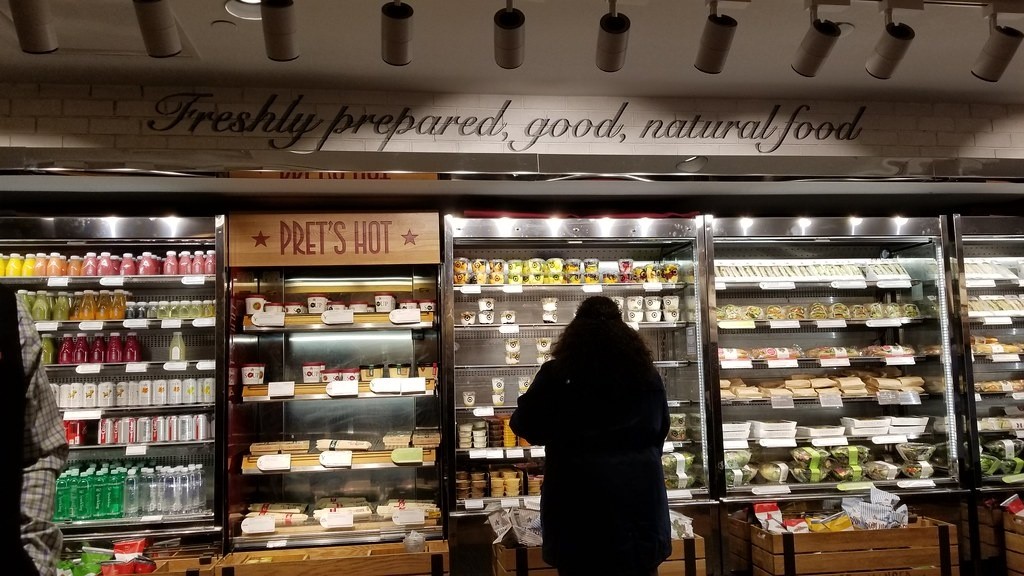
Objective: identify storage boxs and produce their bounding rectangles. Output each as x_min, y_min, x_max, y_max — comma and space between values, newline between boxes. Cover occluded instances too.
492, 533, 706, 576
961, 501, 1024, 576
214, 540, 450, 576
728, 512, 960, 576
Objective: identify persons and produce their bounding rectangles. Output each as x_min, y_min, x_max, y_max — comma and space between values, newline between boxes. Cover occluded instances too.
509, 296, 673, 576
0, 283, 68, 576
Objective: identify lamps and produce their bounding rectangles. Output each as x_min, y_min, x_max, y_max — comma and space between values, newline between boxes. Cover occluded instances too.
694, 0, 738, 75
596, 0, 632, 73
865, 8, 915, 80
132, 0, 182, 59
260, 0, 300, 63
790, 7, 841, 77
381, 0, 415, 67
971, 14, 1024, 83
494, 0, 526, 70
8, 0, 60, 55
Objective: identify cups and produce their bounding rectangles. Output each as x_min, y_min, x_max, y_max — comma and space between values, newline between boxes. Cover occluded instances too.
454, 256, 680, 408
242, 293, 436, 381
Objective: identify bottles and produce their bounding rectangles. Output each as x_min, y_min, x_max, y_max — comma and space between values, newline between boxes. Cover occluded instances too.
170, 332, 185, 363
51, 460, 205, 523
1, 246, 215, 277
18, 289, 216, 322
40, 332, 139, 366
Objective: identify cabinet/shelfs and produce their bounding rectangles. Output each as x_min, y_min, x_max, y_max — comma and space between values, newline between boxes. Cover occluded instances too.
455, 283, 690, 509
964, 289, 1024, 485
232, 312, 437, 549
0, 274, 215, 528
713, 277, 955, 483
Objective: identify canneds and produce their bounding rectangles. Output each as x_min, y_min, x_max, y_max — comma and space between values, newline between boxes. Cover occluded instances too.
63, 412, 208, 446
49, 377, 216, 409
